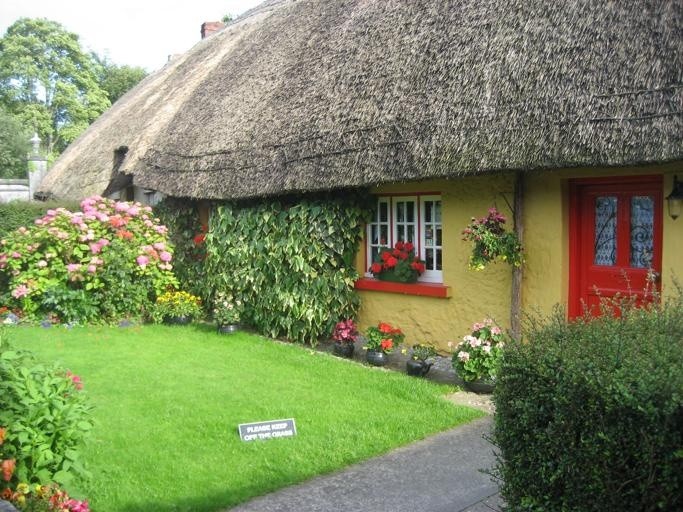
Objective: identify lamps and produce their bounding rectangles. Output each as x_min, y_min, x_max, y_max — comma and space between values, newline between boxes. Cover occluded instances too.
665, 175, 681, 221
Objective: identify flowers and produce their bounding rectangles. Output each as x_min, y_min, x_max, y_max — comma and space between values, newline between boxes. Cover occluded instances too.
148, 289, 202, 326
461, 206, 526, 272
328, 316, 358, 343
208, 290, 242, 326
447, 313, 513, 385
362, 321, 406, 355
401, 337, 439, 361
363, 241, 428, 282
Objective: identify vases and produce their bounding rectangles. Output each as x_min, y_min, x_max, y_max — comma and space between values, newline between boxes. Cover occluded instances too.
367, 350, 386, 364
405, 357, 433, 378
216, 323, 236, 334
467, 381, 498, 393
378, 272, 417, 283
165, 315, 191, 327
333, 340, 353, 357
